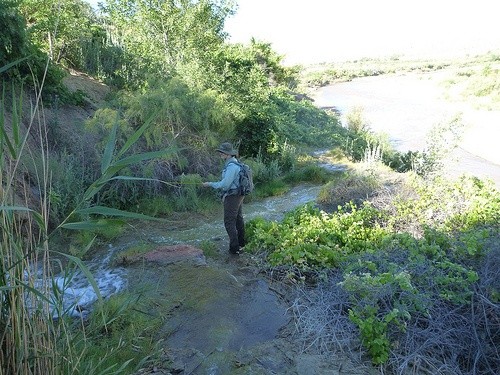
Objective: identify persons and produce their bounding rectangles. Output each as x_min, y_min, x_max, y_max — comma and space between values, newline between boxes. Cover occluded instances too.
202, 143, 246, 255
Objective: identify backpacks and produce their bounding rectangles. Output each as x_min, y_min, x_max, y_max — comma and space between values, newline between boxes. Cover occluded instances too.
226, 161, 254, 196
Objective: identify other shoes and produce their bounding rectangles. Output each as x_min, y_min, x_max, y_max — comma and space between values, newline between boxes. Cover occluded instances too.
229, 248, 242, 254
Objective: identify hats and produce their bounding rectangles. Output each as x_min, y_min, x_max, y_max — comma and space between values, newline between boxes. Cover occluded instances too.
216, 143, 238, 156
